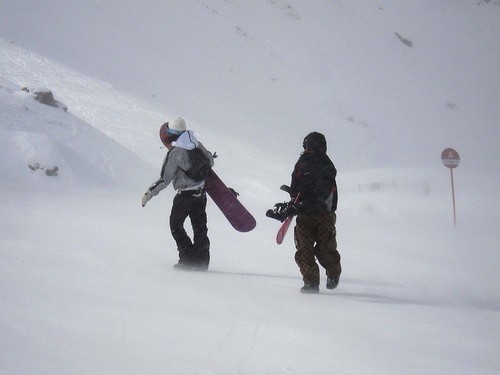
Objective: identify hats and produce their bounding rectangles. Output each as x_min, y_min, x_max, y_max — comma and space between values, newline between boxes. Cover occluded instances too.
168, 116, 186, 135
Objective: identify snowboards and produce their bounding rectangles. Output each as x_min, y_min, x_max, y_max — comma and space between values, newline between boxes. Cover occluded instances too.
204, 168, 256, 232
277, 191, 300, 244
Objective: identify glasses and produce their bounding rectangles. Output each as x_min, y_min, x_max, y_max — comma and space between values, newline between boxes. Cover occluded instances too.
303, 144, 315, 150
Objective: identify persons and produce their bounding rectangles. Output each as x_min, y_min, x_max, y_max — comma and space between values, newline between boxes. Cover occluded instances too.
141, 117, 214, 273
290, 132, 342, 295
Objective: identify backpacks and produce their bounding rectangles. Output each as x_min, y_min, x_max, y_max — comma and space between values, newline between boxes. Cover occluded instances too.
179, 147, 211, 181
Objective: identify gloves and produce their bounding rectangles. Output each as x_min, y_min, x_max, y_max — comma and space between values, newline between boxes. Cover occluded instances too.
141, 190, 153, 207
291, 203, 302, 214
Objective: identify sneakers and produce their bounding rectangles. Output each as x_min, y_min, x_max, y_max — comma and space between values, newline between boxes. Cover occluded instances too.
174, 261, 191, 270
193, 264, 208, 270
326, 266, 341, 289
300, 283, 319, 293
